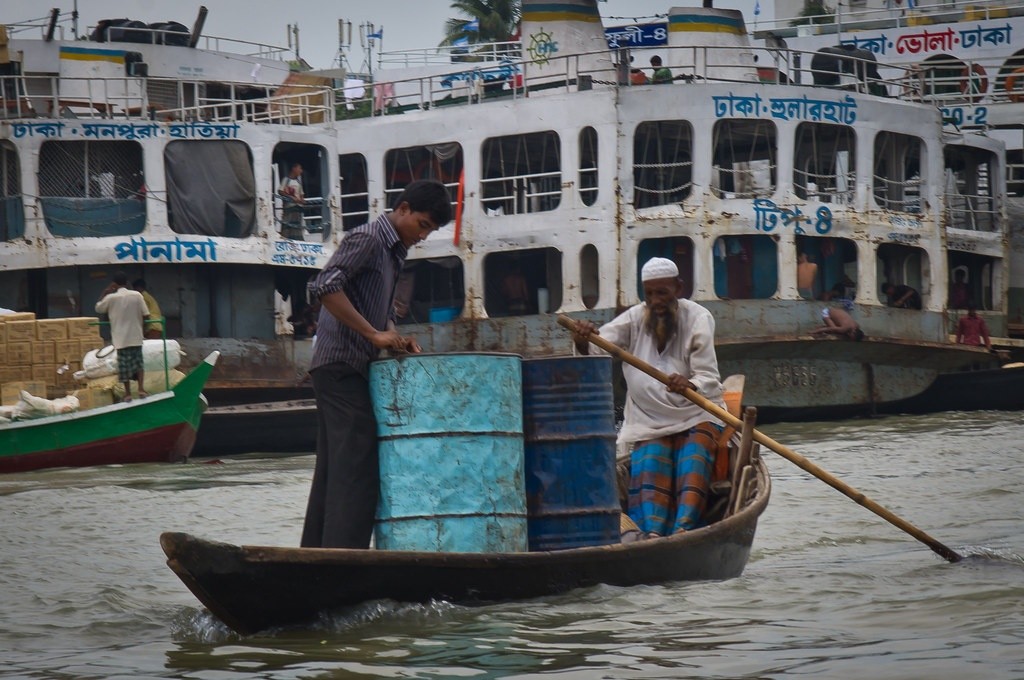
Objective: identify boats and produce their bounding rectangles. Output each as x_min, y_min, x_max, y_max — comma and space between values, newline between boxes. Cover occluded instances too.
0, 348, 223, 477
0, 0, 1023, 462
158, 428, 772, 637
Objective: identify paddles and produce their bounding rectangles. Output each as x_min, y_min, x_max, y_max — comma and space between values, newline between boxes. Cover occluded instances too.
555, 316, 976, 566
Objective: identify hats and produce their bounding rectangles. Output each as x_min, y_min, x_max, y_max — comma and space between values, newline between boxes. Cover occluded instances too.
642, 257, 680, 279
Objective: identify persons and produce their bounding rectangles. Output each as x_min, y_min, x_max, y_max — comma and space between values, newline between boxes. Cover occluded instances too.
948, 269, 975, 308
278, 162, 306, 242
807, 305, 865, 339
650, 56, 672, 83
94, 272, 150, 402
133, 278, 163, 339
956, 302, 993, 352
301, 181, 454, 549
817, 283, 846, 300
881, 282, 922, 311
573, 257, 726, 539
503, 264, 531, 317
798, 250, 819, 300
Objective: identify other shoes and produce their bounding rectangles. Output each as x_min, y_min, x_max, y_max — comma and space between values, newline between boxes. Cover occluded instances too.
139, 392, 152, 399
118, 395, 131, 403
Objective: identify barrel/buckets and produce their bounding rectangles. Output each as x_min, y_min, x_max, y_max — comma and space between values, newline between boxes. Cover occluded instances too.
519, 354, 622, 554
365, 351, 530, 555
